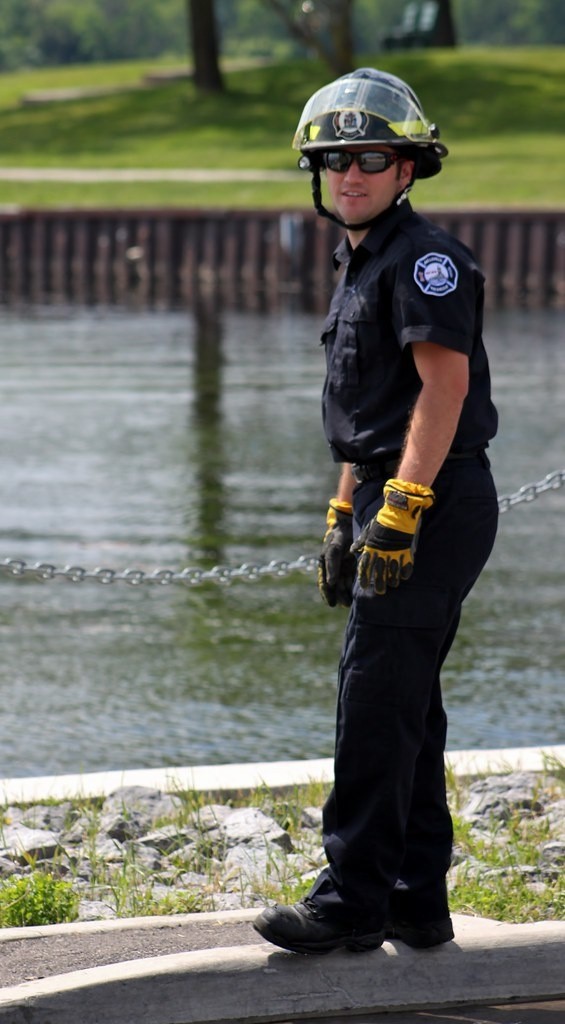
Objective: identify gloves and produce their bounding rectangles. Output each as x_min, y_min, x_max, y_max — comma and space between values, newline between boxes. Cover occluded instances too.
349, 478, 436, 595
317, 498, 352, 608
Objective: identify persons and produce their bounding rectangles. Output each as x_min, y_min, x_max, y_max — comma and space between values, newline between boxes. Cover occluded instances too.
252, 69, 500, 955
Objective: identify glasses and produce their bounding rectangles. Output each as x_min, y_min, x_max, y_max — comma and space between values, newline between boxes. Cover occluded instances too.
321, 149, 401, 175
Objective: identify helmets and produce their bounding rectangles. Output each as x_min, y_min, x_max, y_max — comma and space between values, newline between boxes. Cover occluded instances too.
291, 67, 441, 179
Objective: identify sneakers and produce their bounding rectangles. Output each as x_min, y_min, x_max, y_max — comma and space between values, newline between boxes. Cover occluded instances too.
251, 897, 387, 956
386, 917, 456, 950
